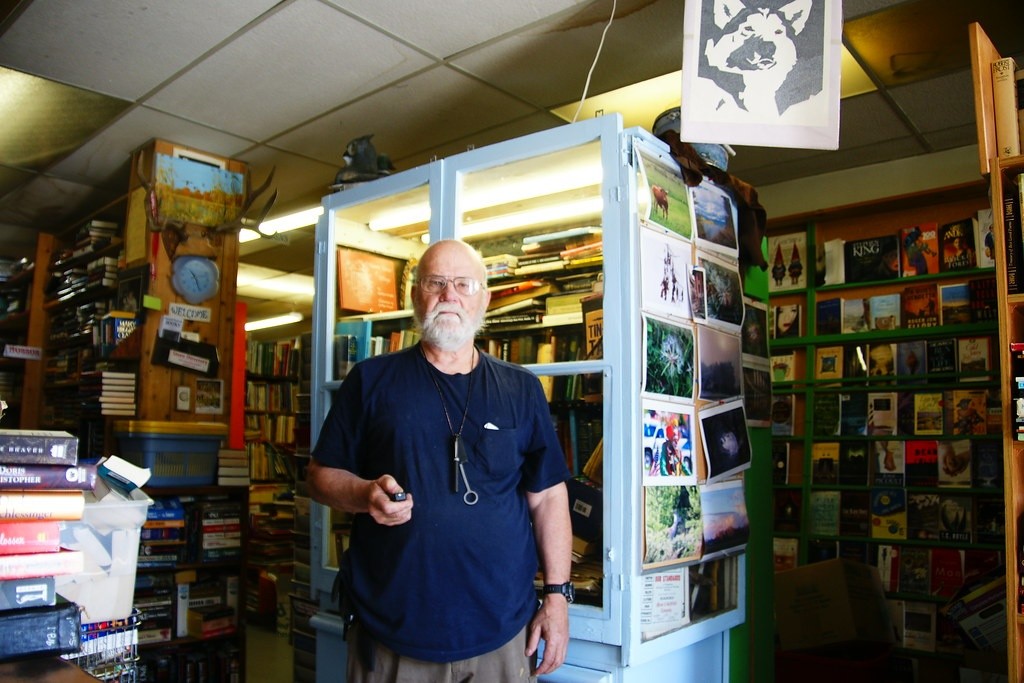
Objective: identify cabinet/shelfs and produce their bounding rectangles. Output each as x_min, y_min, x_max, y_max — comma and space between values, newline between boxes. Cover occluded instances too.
0, 114, 1023, 683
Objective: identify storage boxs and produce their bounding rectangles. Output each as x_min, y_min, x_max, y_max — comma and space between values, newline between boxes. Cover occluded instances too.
43, 486, 155, 619
116, 432, 221, 485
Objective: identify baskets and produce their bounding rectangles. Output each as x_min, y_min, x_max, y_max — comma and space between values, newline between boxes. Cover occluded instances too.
109, 417, 227, 489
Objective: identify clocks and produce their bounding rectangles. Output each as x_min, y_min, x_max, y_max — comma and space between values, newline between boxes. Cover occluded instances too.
171, 257, 220, 304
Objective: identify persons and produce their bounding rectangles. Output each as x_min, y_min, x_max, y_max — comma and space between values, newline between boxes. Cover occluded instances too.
660, 425, 682, 476
307, 239, 573, 683
775, 305, 799, 338
877, 240, 897, 275
953, 238, 971, 263
873, 441, 896, 474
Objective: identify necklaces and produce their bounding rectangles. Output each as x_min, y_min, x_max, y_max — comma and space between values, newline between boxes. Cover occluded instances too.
421, 344, 478, 505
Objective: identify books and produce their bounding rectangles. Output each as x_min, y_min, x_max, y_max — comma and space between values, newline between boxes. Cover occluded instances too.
690, 557, 737, 615
0, 218, 137, 417
247, 333, 311, 620
991, 57, 1024, 158
334, 225, 602, 602
0, 429, 250, 683
772, 173, 1023, 683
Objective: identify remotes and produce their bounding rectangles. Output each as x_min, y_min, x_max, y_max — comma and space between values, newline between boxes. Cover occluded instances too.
391, 493, 406, 501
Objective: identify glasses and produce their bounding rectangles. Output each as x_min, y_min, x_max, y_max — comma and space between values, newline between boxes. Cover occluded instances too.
414, 275, 487, 296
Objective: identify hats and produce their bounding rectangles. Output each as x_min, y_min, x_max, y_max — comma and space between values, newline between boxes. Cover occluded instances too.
956, 398, 972, 407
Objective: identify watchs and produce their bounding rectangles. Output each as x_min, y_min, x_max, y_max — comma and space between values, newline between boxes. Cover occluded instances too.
543, 582, 574, 603
